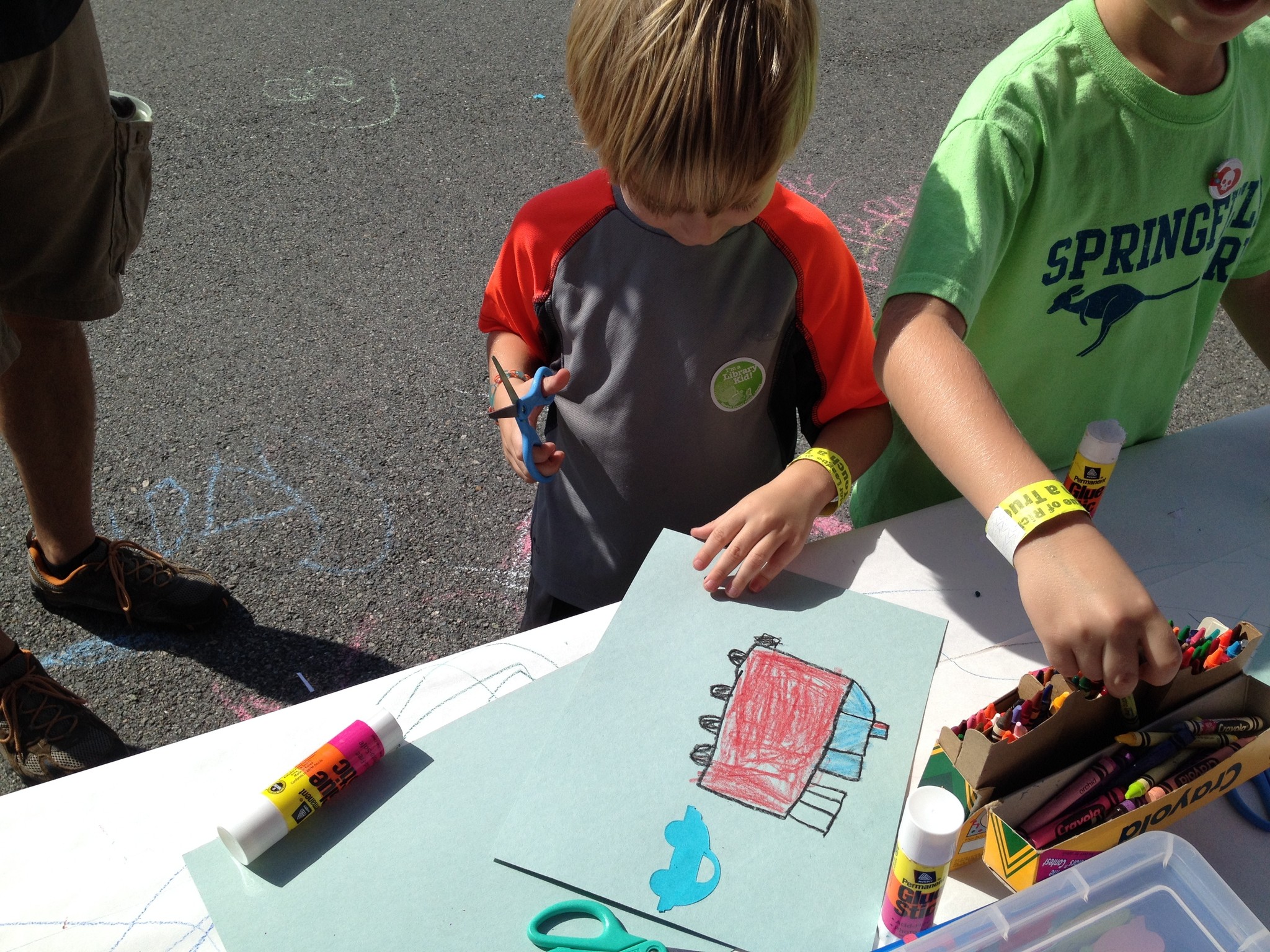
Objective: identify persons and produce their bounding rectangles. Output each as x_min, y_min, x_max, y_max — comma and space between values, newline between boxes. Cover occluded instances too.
851, 0, 1270, 699
0, 1, 228, 786
479, 0, 895, 635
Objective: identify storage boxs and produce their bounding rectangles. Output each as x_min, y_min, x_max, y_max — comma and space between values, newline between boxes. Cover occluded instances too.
869, 827, 1270, 952
917, 620, 1270, 900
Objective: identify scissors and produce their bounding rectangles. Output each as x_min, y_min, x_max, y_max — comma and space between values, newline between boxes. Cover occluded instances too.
1224, 768, 1270, 831
487, 356, 555, 483
528, 899, 704, 952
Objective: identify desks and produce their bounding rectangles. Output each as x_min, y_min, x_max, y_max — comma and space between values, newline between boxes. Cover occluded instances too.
0, 400, 1270, 952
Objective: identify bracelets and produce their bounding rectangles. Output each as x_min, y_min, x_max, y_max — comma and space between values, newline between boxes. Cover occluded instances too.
487, 370, 530, 429
784, 446, 853, 519
985, 480, 1092, 568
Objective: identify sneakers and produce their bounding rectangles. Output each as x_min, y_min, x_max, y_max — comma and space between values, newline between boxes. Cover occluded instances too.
0, 647, 129, 786
24, 524, 221, 624
